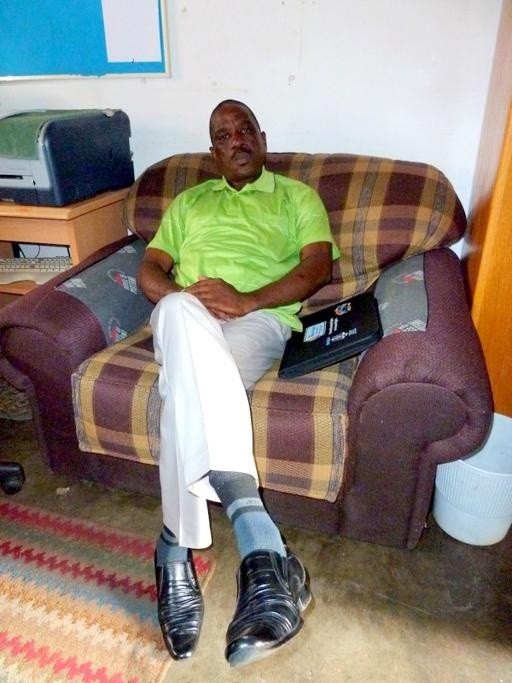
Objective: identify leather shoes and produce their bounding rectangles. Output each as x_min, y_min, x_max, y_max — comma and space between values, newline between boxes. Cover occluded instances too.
225, 543, 311, 668
154, 544, 204, 660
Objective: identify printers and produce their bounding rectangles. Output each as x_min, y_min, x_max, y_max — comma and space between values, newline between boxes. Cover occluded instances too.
0, 109, 134, 208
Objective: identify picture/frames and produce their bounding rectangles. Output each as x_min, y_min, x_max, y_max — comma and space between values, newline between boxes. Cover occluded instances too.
0, 0, 172, 83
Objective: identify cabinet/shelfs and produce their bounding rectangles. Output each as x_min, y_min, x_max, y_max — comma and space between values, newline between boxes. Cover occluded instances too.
0, 183, 133, 309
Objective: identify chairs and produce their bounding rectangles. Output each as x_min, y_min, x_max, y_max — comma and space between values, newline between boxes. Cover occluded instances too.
0, 151, 494, 553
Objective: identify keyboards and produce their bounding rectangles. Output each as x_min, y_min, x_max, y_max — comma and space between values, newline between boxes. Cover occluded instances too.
0, 256, 70, 285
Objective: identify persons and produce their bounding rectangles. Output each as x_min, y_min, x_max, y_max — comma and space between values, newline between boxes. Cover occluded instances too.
138, 98, 340, 670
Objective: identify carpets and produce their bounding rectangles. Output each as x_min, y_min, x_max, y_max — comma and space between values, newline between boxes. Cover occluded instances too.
0, 494, 216, 683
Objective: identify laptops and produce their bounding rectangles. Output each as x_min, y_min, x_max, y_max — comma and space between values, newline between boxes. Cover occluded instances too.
277, 291, 382, 380
